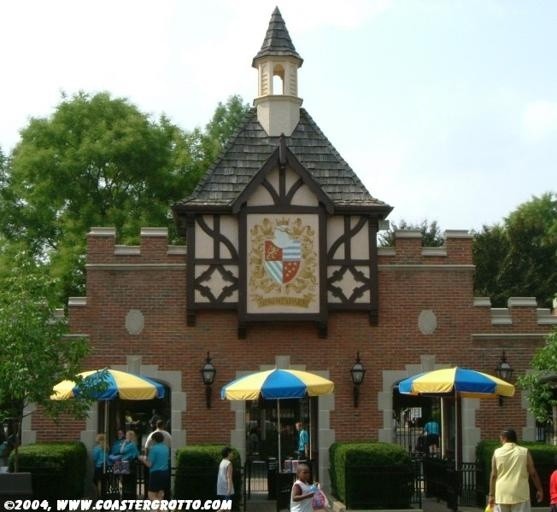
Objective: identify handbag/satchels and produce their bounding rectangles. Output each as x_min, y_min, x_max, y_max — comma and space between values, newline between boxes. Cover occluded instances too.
112, 460, 131, 475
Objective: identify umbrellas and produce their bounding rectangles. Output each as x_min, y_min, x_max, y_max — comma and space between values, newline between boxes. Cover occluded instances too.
396, 364, 516, 471
47, 364, 165, 472
219, 362, 335, 471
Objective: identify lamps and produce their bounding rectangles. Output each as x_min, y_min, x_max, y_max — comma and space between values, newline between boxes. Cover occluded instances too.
351, 352, 367, 407
201, 351, 216, 407
494, 349, 514, 406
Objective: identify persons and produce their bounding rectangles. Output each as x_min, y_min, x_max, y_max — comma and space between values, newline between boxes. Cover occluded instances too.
549, 469, 556, 511
216, 445, 241, 511
392, 409, 398, 431
248, 422, 260, 453
293, 421, 309, 460
420, 416, 440, 457
288, 462, 324, 511
91, 407, 172, 511
487, 427, 543, 511
